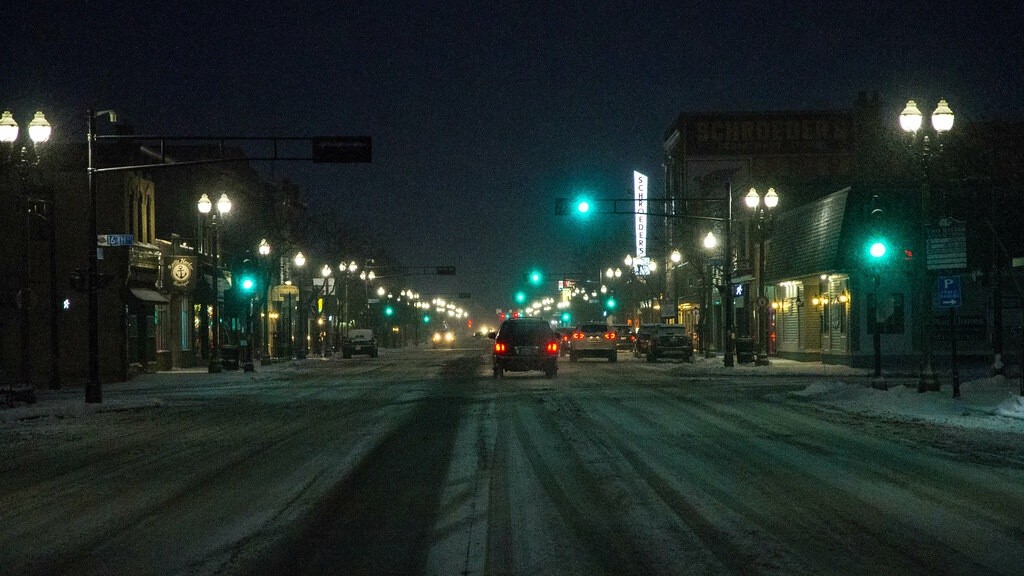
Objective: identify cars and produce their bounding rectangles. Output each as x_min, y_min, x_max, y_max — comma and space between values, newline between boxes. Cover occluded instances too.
612, 322, 694, 363
488, 317, 576, 379
343, 329, 379, 360
569, 322, 617, 363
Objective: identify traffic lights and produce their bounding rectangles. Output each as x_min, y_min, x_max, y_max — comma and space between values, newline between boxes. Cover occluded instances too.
554, 198, 595, 215
865, 207, 892, 265
239, 254, 258, 293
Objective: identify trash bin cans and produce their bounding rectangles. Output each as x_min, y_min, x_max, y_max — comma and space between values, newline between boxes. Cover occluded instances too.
737, 338, 755, 363
222, 346, 241, 370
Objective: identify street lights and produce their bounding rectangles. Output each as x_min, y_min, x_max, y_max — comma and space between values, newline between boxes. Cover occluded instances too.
339, 261, 358, 337
744, 187, 779, 367
899, 100, 956, 394
294, 251, 306, 360
671, 249, 681, 325
606, 268, 622, 324
359, 271, 375, 329
321, 264, 332, 357
259, 239, 271, 366
198, 192, 234, 374
0, 109, 52, 388
624, 254, 644, 326
703, 231, 718, 358
